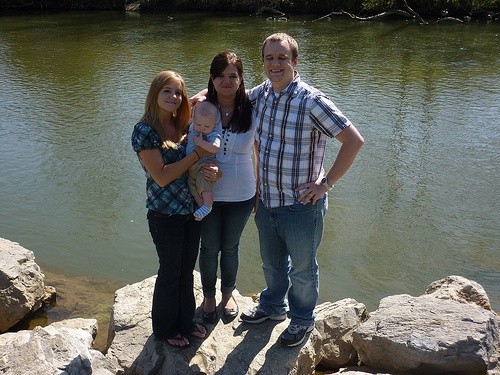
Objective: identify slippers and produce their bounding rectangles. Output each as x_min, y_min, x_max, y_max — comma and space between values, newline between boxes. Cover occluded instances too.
164, 332, 191, 349
188, 321, 209, 338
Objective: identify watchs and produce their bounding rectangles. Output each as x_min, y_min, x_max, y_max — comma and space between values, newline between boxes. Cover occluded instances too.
320, 177, 333, 192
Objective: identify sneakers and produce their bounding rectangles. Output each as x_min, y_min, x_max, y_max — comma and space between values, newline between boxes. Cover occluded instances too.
279, 322, 314, 347
240, 306, 286, 324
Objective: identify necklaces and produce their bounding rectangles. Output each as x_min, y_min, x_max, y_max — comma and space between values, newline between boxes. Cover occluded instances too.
217, 103, 235, 117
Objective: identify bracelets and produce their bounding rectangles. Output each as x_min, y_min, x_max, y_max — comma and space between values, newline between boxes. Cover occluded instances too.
192, 149, 200, 160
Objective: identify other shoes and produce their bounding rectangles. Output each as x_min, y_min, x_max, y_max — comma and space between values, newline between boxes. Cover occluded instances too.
201, 299, 218, 324
221, 295, 239, 318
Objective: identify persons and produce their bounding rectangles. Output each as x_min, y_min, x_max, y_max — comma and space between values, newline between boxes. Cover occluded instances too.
186, 31, 365, 349
130, 69, 222, 349
